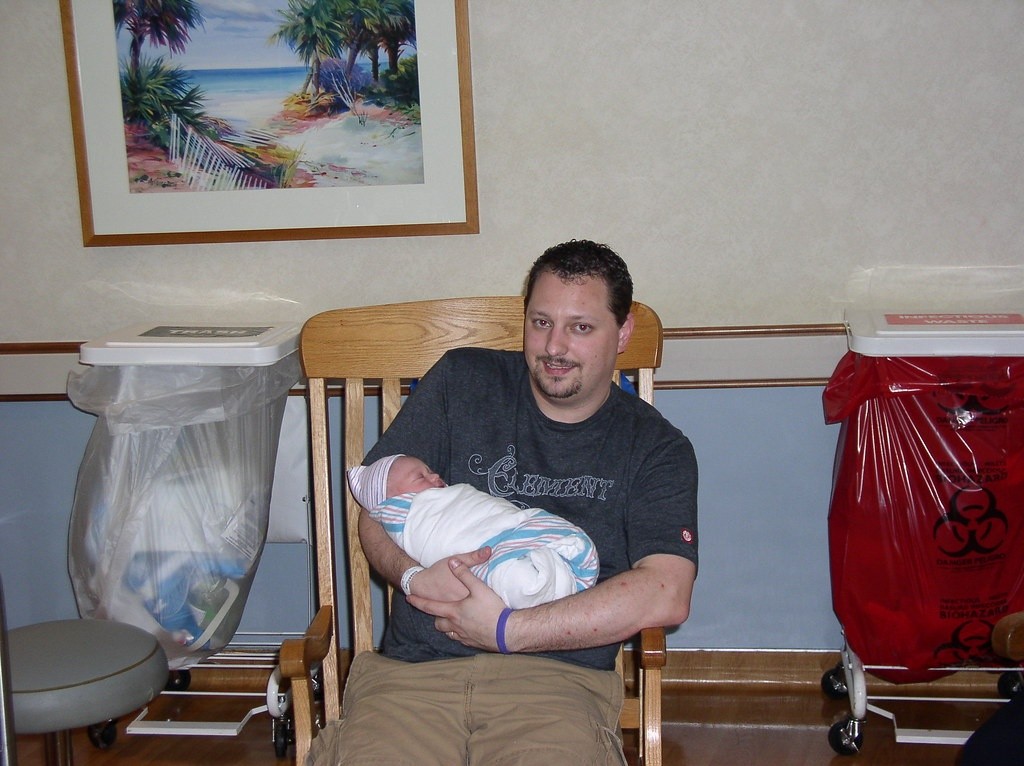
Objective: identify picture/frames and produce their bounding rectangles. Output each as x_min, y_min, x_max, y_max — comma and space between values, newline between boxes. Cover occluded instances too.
60, 0, 480, 247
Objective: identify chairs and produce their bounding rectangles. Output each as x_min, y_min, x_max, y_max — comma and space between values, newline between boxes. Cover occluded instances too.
277, 294, 666, 766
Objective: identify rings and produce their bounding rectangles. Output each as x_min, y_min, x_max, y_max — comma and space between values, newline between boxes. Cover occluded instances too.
449, 631, 454, 639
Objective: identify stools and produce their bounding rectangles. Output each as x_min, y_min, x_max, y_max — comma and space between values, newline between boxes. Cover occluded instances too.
6, 617, 169, 766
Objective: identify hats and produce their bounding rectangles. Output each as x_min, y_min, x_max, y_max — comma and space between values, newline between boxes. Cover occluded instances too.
346, 453, 406, 512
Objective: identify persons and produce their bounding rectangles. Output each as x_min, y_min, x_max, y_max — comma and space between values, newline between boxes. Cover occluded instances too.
307, 240, 699, 766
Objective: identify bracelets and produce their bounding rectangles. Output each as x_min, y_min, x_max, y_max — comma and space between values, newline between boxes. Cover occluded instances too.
400, 565, 423, 594
496, 606, 514, 654
